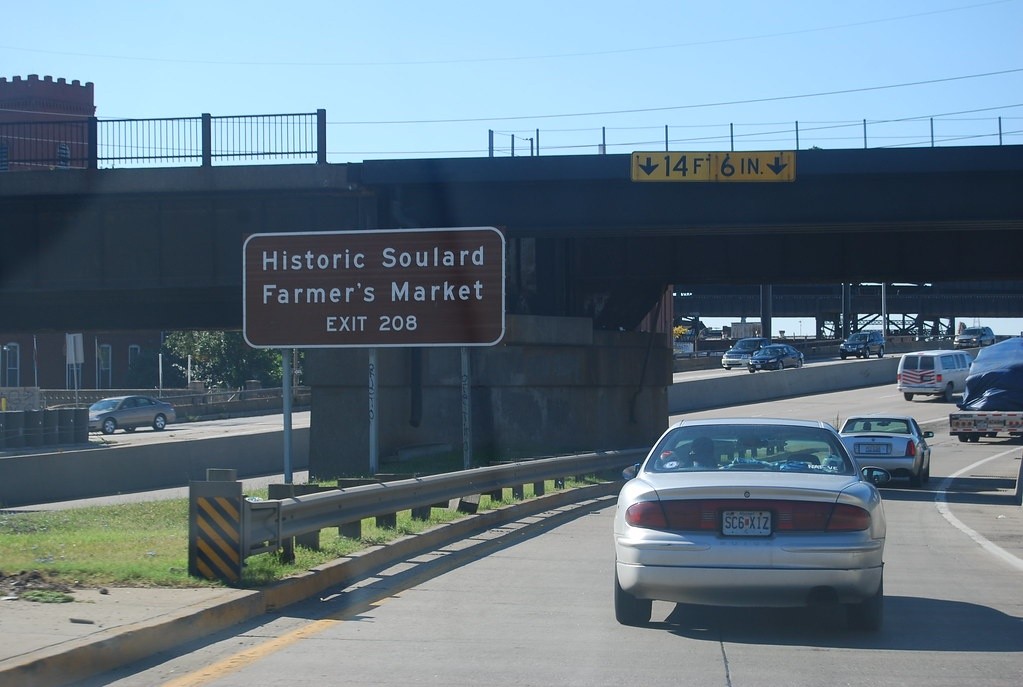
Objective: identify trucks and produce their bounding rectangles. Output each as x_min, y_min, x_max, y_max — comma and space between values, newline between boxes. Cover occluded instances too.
948, 336, 1023, 444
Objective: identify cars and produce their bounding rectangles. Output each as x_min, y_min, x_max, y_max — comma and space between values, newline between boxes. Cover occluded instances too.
838, 330, 885, 360
827, 414, 935, 489
747, 344, 804, 373
953, 326, 997, 350
721, 336, 771, 370
88, 394, 176, 436
613, 416, 891, 633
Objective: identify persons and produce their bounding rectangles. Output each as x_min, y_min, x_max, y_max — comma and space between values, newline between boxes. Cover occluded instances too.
689, 437, 714, 468
863, 422, 872, 430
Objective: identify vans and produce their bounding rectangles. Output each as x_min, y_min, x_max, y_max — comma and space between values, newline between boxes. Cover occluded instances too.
896, 349, 975, 403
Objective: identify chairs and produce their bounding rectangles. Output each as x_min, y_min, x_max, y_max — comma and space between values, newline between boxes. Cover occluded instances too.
786, 452, 820, 465
